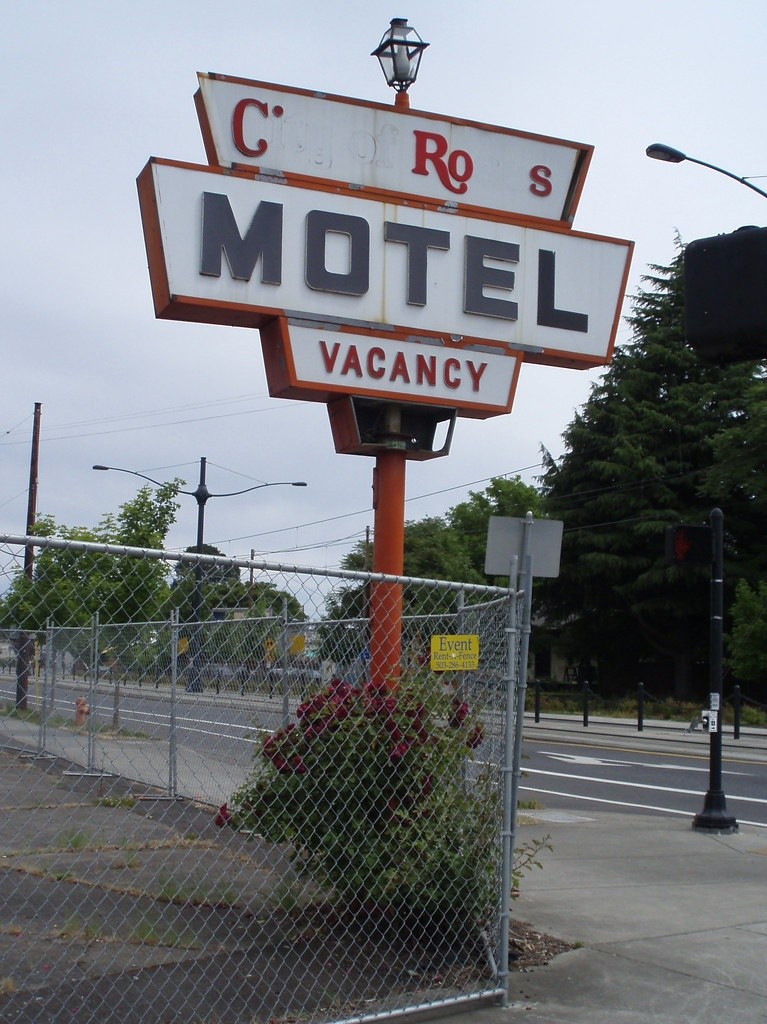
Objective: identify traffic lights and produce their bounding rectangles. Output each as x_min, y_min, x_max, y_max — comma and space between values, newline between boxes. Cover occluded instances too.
664, 521, 717, 568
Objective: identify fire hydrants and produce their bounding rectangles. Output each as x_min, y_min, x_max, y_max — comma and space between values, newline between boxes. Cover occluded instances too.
75, 698, 88, 726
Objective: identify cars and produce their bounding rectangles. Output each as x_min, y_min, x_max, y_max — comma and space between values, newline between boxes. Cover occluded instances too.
198, 662, 320, 690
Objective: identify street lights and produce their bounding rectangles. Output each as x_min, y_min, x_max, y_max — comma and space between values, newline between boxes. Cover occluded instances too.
93, 456, 307, 692
328, 16, 459, 695
